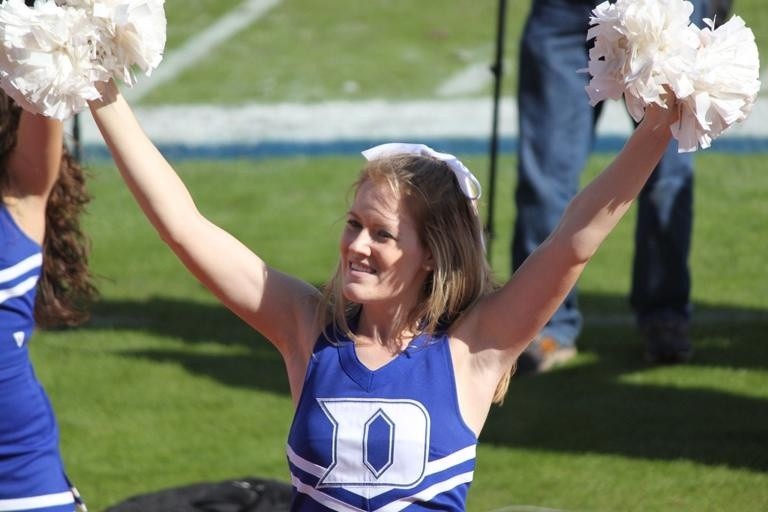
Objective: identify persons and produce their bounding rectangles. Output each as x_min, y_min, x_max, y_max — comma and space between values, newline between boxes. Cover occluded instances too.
512, 0, 733, 374
0, 0, 102, 512
88, 77, 682, 512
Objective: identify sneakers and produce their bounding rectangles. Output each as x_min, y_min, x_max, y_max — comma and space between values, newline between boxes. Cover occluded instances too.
516, 337, 576, 370
643, 320, 690, 363
